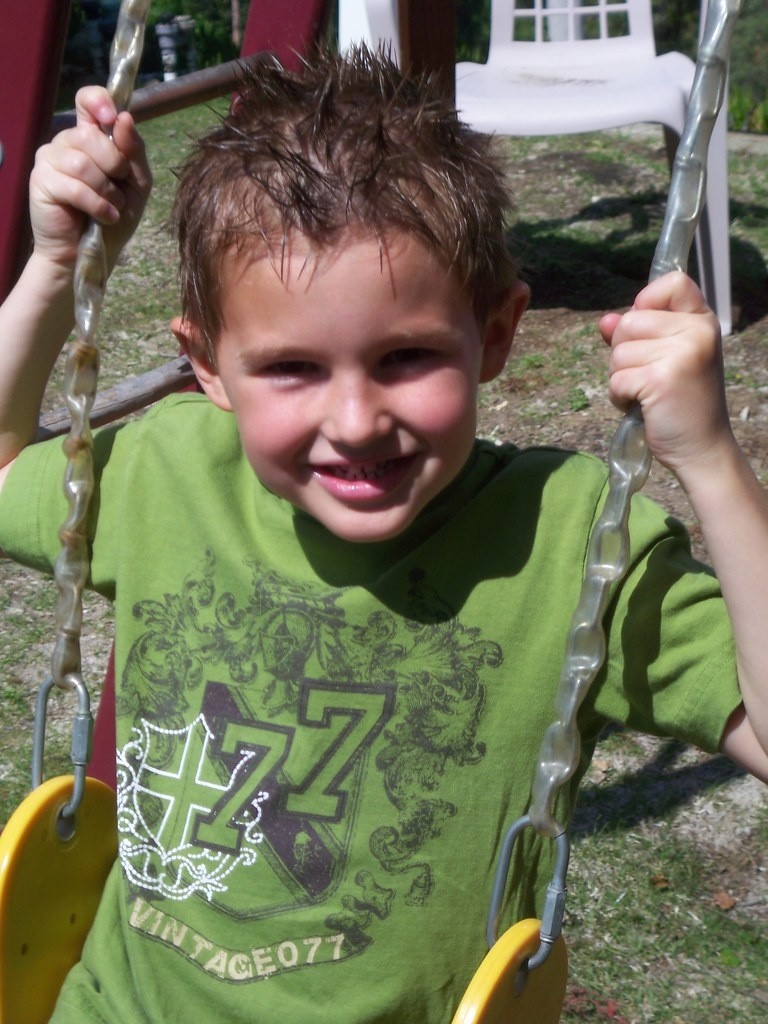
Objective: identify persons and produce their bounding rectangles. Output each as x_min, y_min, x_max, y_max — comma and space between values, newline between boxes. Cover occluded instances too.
0, 31, 768, 1024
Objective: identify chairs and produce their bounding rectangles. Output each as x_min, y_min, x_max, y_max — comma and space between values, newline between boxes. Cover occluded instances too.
333, 0, 732, 338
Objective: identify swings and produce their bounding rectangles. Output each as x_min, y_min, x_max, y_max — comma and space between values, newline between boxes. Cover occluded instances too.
0, 0, 737, 1024
